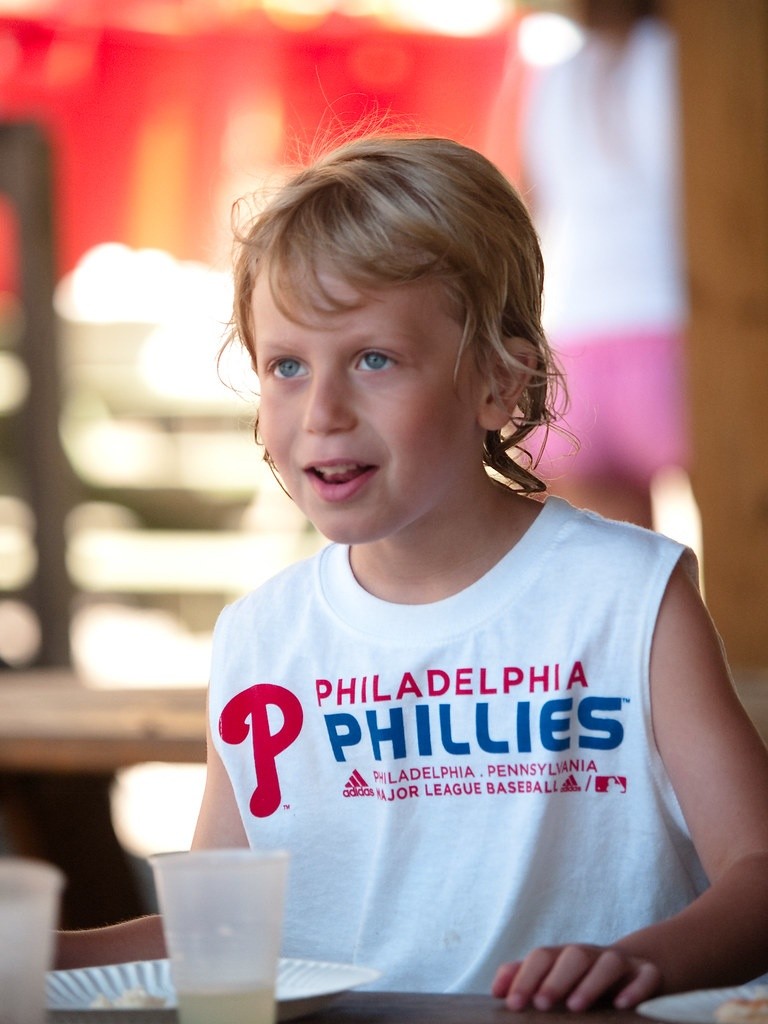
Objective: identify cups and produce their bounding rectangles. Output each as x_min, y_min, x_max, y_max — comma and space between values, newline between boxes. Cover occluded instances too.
0, 856, 69, 1024
144, 847, 286, 1024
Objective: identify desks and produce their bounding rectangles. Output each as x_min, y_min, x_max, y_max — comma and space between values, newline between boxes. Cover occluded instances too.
2, 685, 210, 926
283, 992, 661, 1024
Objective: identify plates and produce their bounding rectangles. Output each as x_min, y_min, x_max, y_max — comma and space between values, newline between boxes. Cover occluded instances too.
42, 953, 378, 1024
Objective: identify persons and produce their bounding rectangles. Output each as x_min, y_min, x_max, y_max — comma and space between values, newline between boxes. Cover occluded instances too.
52, 133, 767, 1013
